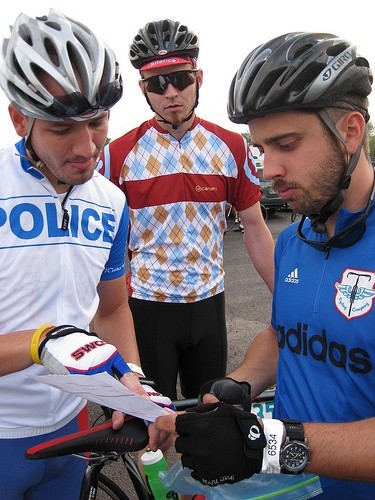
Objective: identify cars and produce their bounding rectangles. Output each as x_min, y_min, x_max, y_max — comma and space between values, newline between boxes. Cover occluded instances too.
255, 165, 286, 215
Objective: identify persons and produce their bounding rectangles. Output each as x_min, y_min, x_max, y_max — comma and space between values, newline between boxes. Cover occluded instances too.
0, 7, 185, 500
92, 19, 275, 416
156, 30, 375, 500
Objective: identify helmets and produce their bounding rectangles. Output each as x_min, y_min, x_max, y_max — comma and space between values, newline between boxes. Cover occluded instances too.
128, 18, 200, 71
0, 5, 123, 122
226, 30, 374, 124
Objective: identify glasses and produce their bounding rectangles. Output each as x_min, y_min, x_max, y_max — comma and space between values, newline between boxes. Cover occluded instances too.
298, 206, 375, 255
140, 66, 196, 94
32, 80, 124, 118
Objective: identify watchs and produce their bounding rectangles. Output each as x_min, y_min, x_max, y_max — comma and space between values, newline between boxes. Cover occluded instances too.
278, 418, 311, 476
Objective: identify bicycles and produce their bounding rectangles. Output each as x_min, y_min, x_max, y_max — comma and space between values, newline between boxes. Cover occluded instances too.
25, 389, 275, 500
225, 202, 268, 232
292, 210, 297, 223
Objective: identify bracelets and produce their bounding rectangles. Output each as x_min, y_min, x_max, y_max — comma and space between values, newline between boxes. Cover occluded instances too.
261, 418, 286, 474
31, 324, 57, 365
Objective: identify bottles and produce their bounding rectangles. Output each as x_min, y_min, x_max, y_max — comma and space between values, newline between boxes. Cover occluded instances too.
140, 448, 180, 500
